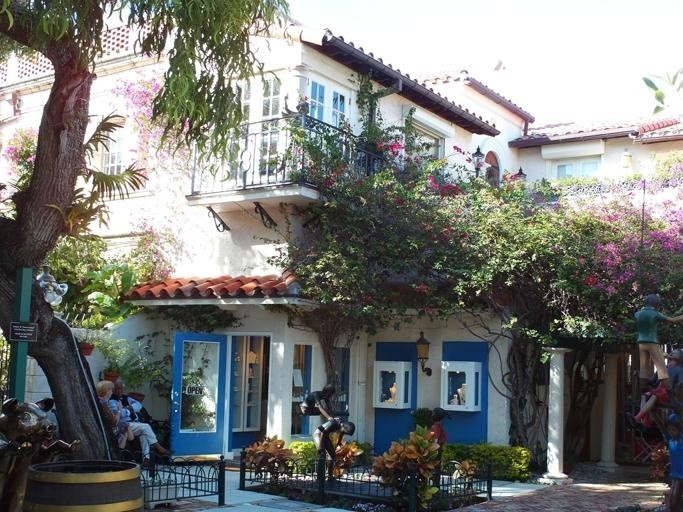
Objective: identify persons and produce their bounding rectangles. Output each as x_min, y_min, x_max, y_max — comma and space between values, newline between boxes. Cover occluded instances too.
626, 348, 682, 429
312, 418, 355, 482
429, 406, 446, 486
633, 293, 683, 390
299, 383, 336, 420
112, 380, 168, 470
648, 410, 683, 512
96, 380, 134, 449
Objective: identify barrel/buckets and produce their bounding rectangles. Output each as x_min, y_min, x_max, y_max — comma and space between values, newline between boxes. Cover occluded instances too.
23, 457, 145, 512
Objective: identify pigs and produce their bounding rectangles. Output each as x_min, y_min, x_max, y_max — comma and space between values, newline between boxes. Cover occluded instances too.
0, 397, 80, 511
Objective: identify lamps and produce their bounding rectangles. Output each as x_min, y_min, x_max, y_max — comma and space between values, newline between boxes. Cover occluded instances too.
414, 330, 433, 376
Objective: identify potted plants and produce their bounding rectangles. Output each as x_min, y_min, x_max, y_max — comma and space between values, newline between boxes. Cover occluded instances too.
72, 324, 162, 405
